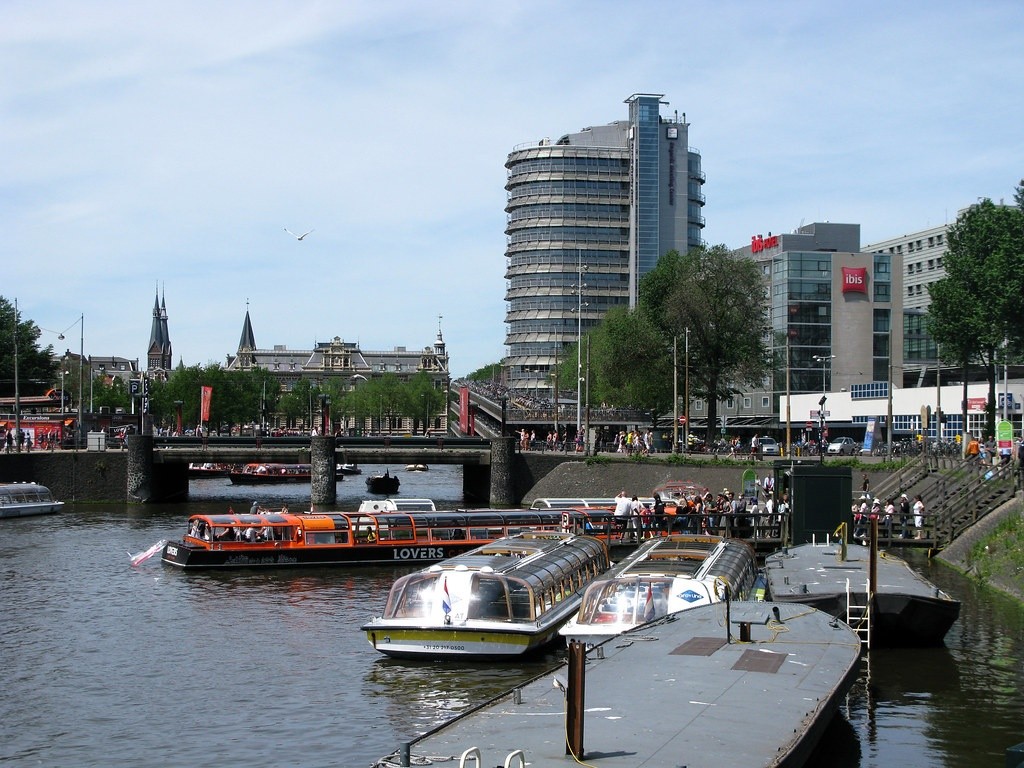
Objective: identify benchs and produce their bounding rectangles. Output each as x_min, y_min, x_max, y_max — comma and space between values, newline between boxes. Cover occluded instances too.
596, 583, 668, 632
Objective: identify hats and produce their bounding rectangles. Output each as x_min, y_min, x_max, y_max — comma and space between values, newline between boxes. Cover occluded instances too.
859, 496, 866, 500
873, 499, 880, 504
901, 494, 908, 500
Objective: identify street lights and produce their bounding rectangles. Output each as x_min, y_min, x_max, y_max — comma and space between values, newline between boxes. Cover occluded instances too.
570, 247, 589, 431
509, 366, 514, 389
550, 373, 555, 407
534, 370, 539, 401
443, 388, 448, 436
813, 355, 838, 426
525, 368, 530, 393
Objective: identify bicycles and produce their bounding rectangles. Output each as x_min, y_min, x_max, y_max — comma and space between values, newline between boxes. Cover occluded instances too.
849, 439, 961, 456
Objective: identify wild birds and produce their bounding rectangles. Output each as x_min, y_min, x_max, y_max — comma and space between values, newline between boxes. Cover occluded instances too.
284, 227, 316, 240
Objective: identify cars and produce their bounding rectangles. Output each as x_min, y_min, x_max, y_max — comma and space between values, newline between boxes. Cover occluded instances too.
827, 437, 856, 456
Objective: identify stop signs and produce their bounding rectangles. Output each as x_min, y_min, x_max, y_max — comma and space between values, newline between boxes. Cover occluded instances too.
679, 416, 686, 424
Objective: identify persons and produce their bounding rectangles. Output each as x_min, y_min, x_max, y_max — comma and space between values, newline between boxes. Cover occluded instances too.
967, 435, 996, 462
688, 430, 694, 455
515, 427, 585, 453
367, 526, 377, 543
195, 501, 290, 542
90, 427, 95, 432
614, 491, 664, 543
859, 474, 869, 495
850, 494, 924, 539
1017, 437, 1024, 447
101, 426, 106, 432
618, 429, 654, 457
726, 436, 741, 459
673, 492, 790, 537
120, 428, 125, 451
312, 426, 319, 437
167, 426, 171, 437
6, 429, 33, 453
746, 433, 760, 461
196, 425, 201, 436
38, 429, 61, 451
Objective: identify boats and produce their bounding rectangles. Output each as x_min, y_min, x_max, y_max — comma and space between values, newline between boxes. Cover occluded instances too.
366, 585, 862, 768
358, 531, 612, 659
364, 468, 400, 493
335, 463, 363, 474
405, 464, 428, 471
558, 533, 768, 650
760, 511, 962, 649
224, 463, 344, 485
0, 481, 66, 518
185, 462, 245, 478
158, 495, 711, 572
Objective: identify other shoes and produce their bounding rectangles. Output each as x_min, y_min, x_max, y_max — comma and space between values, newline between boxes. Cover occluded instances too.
914, 537, 921, 539
860, 534, 866, 537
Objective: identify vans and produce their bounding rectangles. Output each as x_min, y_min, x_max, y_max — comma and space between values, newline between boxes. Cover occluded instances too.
756, 437, 779, 456
